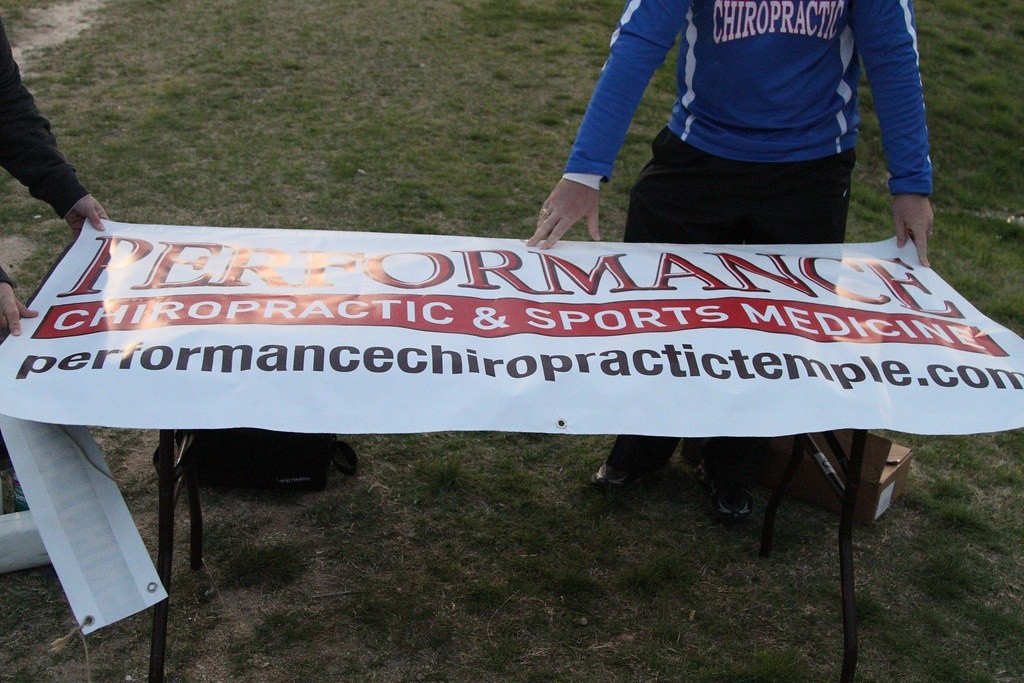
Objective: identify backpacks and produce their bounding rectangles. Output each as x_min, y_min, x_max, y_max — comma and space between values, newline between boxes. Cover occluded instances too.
173, 428, 357, 496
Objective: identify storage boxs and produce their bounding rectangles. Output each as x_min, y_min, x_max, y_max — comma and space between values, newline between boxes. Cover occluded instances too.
767, 428, 912, 528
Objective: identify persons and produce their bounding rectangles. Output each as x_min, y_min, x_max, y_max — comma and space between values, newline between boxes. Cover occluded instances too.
526, 0, 935, 518
1, 18, 111, 512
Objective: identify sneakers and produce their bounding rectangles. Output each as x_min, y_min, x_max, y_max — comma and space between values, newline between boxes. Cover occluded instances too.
709, 482, 753, 523
592, 462, 636, 492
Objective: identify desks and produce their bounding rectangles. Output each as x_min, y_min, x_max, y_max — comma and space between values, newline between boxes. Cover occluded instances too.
0, 212, 1024, 683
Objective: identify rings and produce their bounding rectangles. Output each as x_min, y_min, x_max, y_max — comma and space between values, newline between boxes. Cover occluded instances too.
541, 209, 550, 218
